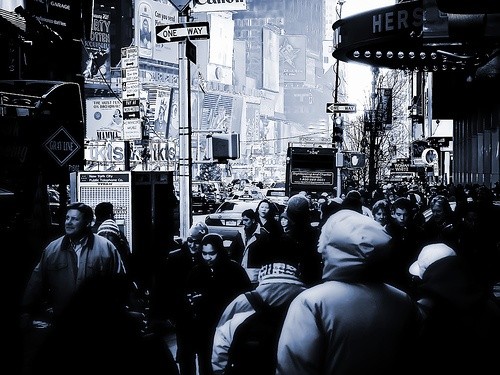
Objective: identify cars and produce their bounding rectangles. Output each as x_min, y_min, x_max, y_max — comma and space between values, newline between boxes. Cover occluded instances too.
191, 182, 287, 240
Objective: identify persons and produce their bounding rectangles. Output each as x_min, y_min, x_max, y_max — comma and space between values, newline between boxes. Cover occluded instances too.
167, 180, 500, 375
29, 202, 128, 375
101, 109, 123, 130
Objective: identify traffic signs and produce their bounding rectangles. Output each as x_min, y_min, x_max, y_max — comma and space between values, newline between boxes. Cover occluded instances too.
326, 102, 356, 113
156, 22, 210, 43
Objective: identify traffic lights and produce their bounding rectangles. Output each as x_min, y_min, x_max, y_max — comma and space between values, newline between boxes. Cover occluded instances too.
334, 117, 343, 143
